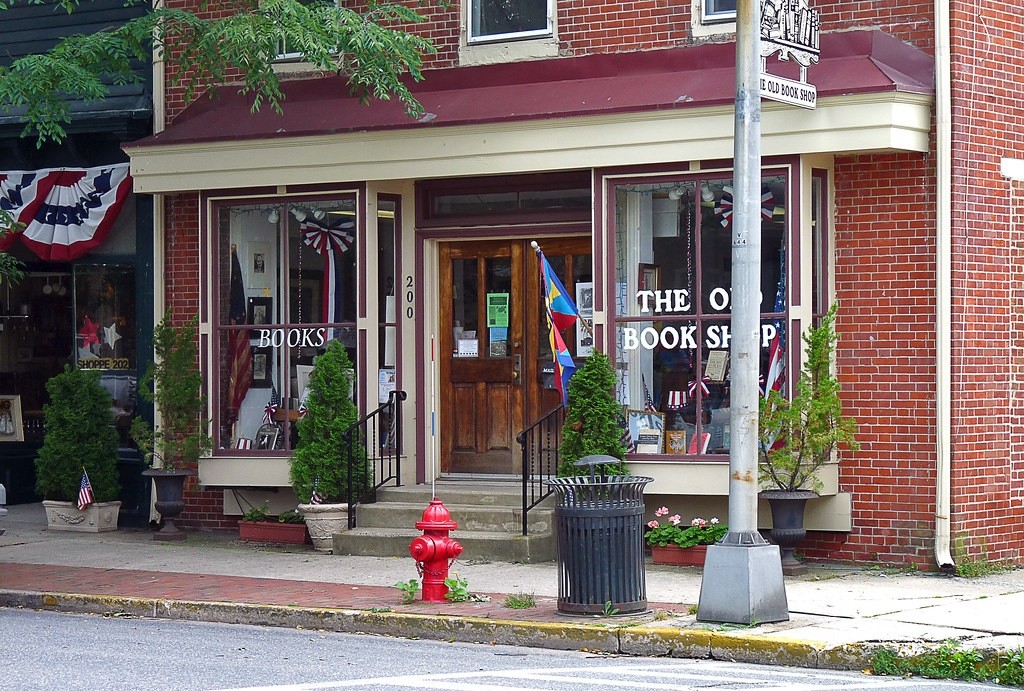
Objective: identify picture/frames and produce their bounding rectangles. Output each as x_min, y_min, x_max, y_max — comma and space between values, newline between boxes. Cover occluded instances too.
638, 263, 662, 306
0, 394, 24, 442
311, 211, 326, 221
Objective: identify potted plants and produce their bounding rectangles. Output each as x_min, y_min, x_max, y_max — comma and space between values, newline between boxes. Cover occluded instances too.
128, 303, 215, 541
288, 336, 374, 551
645, 513, 732, 568
36, 362, 122, 532
237, 503, 313, 545
757, 297, 862, 576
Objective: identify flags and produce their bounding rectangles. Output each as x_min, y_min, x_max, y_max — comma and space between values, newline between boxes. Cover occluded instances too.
758, 222, 790, 463
223, 250, 253, 437
538, 250, 579, 415
77, 473, 93, 511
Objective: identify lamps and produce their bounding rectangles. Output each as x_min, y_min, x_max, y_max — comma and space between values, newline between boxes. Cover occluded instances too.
668, 186, 685, 201
290, 206, 307, 222
267, 208, 279, 224
701, 185, 715, 202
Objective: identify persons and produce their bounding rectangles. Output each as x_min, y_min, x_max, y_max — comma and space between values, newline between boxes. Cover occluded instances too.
254, 254, 264, 273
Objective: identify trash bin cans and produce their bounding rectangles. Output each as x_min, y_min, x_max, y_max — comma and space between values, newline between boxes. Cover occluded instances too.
542, 454, 655, 617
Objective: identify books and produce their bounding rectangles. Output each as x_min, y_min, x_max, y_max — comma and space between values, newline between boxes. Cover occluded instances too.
666, 430, 687, 454
687, 432, 711, 454
